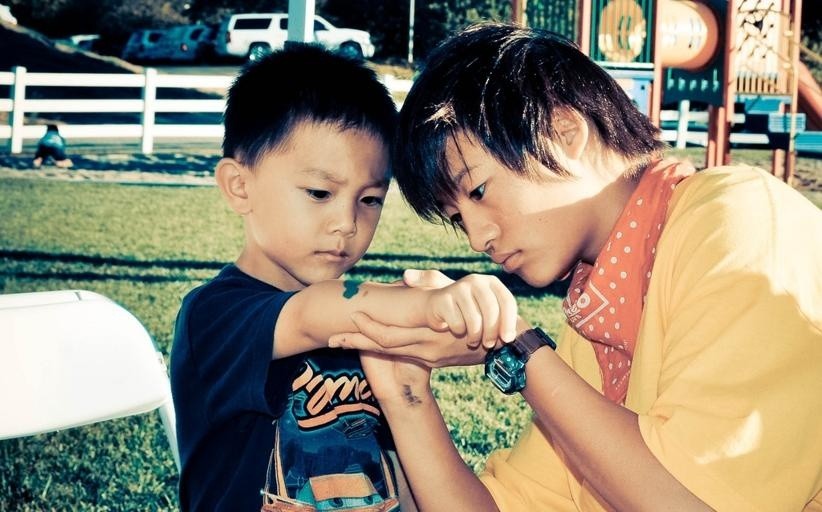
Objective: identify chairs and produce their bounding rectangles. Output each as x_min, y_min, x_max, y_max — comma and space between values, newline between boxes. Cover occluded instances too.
0, 289, 186, 512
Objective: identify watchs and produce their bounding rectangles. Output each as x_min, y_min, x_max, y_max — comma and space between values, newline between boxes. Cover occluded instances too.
483, 327, 556, 396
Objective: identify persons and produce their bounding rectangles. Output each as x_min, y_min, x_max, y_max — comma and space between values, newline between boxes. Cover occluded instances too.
172, 42, 517, 511
33, 125, 74, 168
327, 21, 822, 512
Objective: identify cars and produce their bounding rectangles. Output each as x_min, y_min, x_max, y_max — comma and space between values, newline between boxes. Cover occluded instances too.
123, 12, 376, 62
